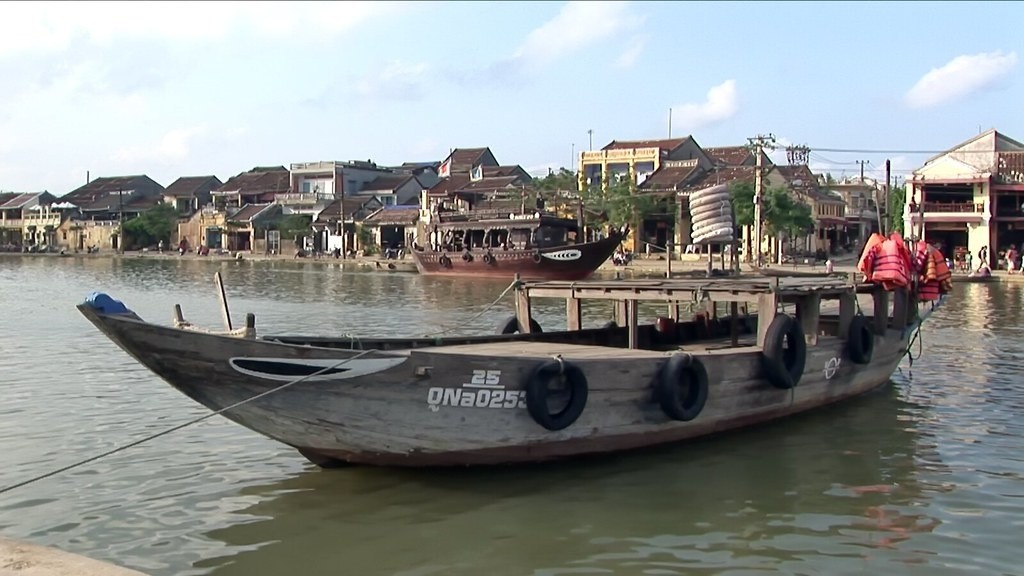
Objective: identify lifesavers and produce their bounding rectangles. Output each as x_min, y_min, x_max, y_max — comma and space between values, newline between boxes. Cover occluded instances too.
658, 353, 710, 421
528, 360, 590, 431
439, 256, 449, 267
495, 315, 544, 337
461, 253, 471, 262
763, 314, 808, 389
483, 254, 492, 265
532, 254, 542, 265
847, 314, 873, 366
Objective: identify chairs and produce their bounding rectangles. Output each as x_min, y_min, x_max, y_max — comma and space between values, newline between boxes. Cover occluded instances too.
724, 325, 751, 338
676, 322, 701, 344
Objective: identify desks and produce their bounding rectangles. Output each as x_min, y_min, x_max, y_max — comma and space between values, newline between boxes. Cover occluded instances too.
666, 334, 787, 351
953, 249, 971, 270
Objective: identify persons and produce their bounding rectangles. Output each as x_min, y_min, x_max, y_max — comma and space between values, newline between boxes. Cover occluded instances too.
933, 243, 1024, 275
178, 235, 210, 257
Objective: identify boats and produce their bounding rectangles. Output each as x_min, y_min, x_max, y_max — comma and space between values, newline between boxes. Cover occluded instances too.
407, 183, 632, 282
74, 239, 952, 470
